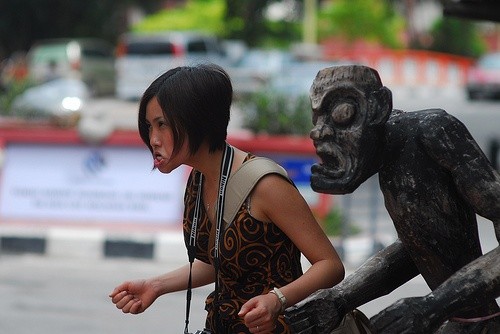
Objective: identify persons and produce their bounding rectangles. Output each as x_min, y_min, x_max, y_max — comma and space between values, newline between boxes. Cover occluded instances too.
109, 62, 345, 334
283, 64, 500, 334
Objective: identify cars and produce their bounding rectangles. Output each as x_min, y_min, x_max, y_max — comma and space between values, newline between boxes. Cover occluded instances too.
467, 54, 500, 100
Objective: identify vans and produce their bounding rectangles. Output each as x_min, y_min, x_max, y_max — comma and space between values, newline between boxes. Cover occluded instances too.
118, 32, 226, 105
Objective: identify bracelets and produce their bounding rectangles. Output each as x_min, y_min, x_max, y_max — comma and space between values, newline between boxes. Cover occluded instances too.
268, 285, 287, 314
